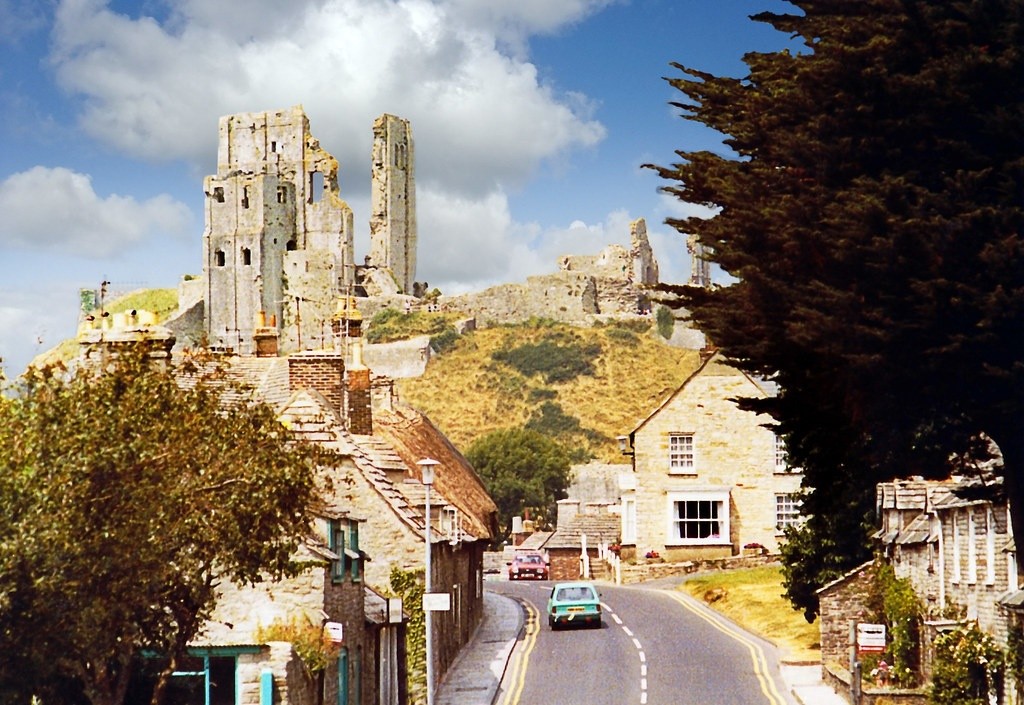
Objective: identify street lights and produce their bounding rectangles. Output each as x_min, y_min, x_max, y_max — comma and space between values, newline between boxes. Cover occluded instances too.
415, 456, 441, 705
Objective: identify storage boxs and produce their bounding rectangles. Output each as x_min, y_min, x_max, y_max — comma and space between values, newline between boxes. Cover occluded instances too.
857, 623, 887, 648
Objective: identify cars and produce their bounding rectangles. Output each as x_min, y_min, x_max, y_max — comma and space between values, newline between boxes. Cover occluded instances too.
547, 583, 602, 630
507, 552, 550, 580
